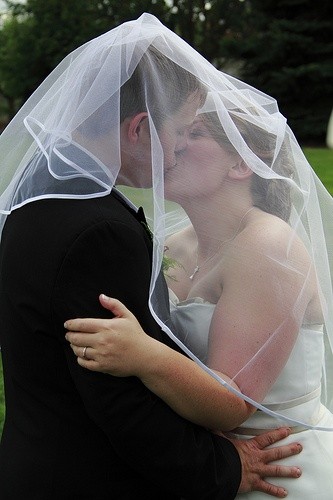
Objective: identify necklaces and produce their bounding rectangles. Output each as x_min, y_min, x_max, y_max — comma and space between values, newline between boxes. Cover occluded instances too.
190, 207, 255, 279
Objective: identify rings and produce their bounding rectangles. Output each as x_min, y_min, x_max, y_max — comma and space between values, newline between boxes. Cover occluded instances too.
82, 346, 89, 361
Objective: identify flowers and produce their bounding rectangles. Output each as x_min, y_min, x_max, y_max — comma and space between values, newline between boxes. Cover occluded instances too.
142, 221, 188, 282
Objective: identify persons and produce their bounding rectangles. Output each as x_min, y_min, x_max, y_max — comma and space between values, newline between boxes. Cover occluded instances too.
65, 105, 333, 500
0, 35, 303, 500
